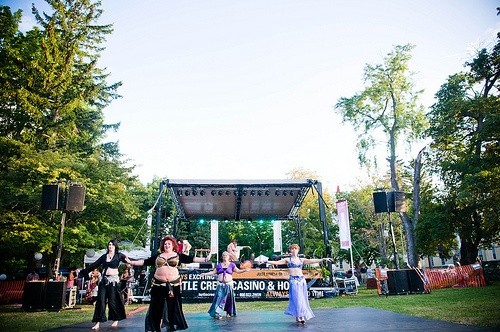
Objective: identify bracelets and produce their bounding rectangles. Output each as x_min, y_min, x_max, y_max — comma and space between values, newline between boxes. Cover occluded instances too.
264, 261, 268, 264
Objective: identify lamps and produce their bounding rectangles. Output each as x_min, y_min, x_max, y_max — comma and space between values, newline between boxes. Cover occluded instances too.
234, 191, 239, 197
184, 190, 190, 196
282, 190, 288, 197
177, 189, 183, 196
257, 190, 263, 197
192, 190, 197, 196
200, 190, 206, 196
295, 200, 301, 207
298, 190, 303, 197
211, 190, 216, 196
218, 190, 223, 196
248, 217, 252, 224
290, 190, 294, 196
275, 190, 280, 197
243, 190, 249, 196
223, 216, 228, 223
250, 190, 255, 197
226, 191, 230, 196
265, 190, 272, 196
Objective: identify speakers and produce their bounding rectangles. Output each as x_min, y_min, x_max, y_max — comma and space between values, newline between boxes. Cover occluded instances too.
406, 269, 425, 293
23, 281, 45, 311
41, 185, 60, 210
388, 192, 406, 212
387, 270, 409, 294
66, 184, 85, 211
44, 281, 65, 310
200, 262, 212, 268
234, 261, 240, 269
373, 192, 388, 213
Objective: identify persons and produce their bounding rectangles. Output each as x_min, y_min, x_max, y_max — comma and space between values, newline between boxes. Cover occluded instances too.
258, 243, 334, 324
227, 240, 239, 261
72, 239, 132, 331
124, 236, 212, 332
66, 267, 77, 286
121, 264, 147, 305
198, 250, 252, 320
83, 268, 102, 305
455, 256, 466, 287
25, 269, 39, 282
182, 237, 192, 268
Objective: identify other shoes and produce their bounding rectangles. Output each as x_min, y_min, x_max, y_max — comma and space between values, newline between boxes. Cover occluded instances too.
301, 320, 305, 325
218, 316, 222, 320
226, 313, 231, 317
296, 318, 302, 322
92, 325, 99, 330
112, 322, 117, 327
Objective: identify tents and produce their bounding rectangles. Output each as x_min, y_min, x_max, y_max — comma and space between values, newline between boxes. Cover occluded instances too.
80, 238, 151, 304
